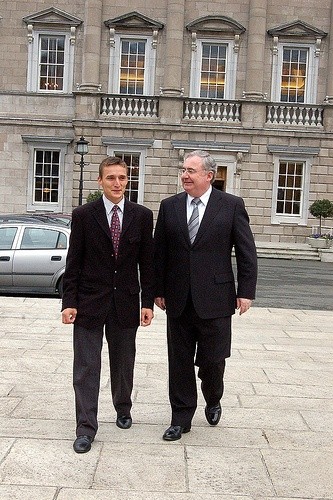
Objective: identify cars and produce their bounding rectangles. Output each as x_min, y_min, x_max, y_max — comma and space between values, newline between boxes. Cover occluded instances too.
0, 212, 72, 300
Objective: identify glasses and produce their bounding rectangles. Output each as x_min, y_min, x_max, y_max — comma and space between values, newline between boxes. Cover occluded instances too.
180, 168, 206, 176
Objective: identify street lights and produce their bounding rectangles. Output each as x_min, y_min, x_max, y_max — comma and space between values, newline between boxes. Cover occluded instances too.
75, 135, 90, 207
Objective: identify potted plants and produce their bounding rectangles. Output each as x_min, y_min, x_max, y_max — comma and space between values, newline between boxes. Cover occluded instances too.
307, 198, 333, 248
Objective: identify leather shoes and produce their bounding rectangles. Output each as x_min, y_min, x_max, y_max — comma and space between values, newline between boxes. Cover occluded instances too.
205, 401, 222, 425
116, 411, 132, 429
163, 423, 191, 441
74, 435, 95, 454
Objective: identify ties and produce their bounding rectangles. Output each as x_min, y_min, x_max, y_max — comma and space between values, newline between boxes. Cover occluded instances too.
110, 205, 121, 259
188, 199, 202, 244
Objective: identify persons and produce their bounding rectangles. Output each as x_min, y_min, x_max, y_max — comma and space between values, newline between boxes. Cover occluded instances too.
153, 150, 258, 441
61, 157, 154, 453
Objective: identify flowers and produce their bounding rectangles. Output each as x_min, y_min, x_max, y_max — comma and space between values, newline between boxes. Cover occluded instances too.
307, 233, 333, 239
